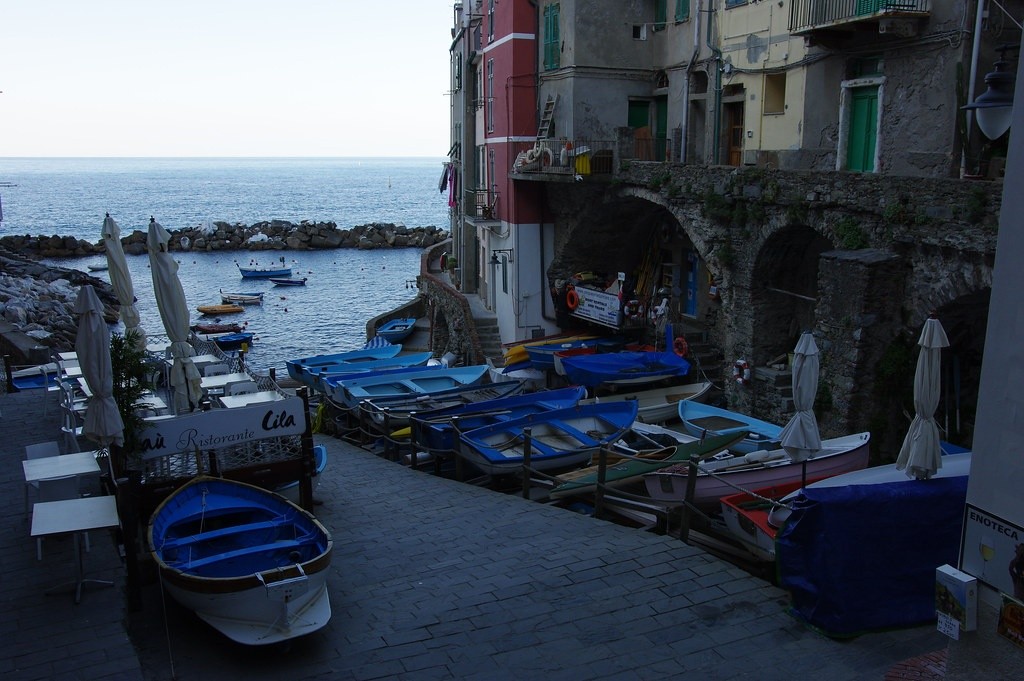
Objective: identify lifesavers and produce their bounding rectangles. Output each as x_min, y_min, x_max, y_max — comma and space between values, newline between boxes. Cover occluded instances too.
732, 359, 751, 385
672, 337, 688, 357
624, 299, 644, 320
566, 289, 579, 310
525, 141, 544, 164
646, 306, 666, 327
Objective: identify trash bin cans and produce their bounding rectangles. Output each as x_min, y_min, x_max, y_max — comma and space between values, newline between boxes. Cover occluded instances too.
454, 268, 461, 286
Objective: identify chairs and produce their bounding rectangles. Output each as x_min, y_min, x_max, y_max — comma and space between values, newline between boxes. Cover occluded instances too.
482, 194, 498, 219
26, 346, 259, 460
36, 475, 89, 561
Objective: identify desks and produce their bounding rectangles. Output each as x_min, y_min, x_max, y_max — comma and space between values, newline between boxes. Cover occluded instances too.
31, 496, 120, 604
21, 451, 102, 491
58, 341, 286, 423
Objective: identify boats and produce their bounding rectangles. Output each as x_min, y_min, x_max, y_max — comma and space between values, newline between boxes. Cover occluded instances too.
269, 277, 307, 286
219, 289, 265, 304
11, 370, 79, 391
147, 477, 335, 645
190, 323, 251, 351
197, 304, 245, 314
128, 444, 327, 507
239, 266, 292, 278
284, 336, 968, 641
87, 264, 108, 270
376, 317, 416, 344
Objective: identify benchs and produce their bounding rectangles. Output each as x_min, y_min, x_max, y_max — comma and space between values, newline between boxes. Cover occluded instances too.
489, 413, 512, 421
182, 538, 299, 569
333, 360, 350, 364
401, 381, 426, 393
547, 420, 594, 445
507, 429, 556, 454
164, 521, 285, 549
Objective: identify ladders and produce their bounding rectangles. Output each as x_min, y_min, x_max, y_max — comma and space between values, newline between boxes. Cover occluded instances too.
536, 92, 559, 139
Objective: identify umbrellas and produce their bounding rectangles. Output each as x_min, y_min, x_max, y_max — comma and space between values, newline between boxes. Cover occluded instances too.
894, 316, 950, 480
146, 217, 203, 411
101, 211, 146, 353
72, 284, 126, 448
775, 327, 823, 492
